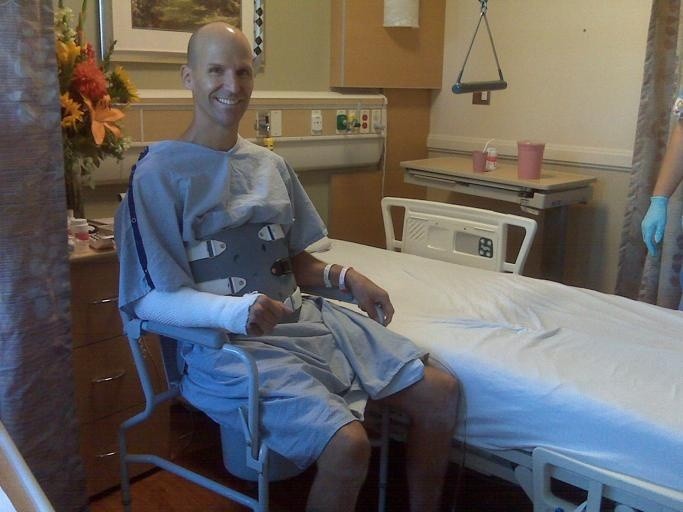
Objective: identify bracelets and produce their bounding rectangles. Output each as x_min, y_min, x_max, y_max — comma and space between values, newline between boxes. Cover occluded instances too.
323, 262, 336, 288
338, 264, 354, 293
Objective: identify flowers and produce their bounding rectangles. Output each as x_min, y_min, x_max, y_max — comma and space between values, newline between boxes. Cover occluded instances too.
52, 0, 141, 179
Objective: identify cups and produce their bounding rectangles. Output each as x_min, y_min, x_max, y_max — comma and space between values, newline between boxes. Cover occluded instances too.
517, 141, 546, 181
472, 151, 488, 173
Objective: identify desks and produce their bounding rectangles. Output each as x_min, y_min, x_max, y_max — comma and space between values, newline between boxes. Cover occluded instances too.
399, 157, 597, 283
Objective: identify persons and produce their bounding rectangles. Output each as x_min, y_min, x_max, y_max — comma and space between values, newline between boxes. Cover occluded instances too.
639, 114, 683, 257
111, 20, 461, 511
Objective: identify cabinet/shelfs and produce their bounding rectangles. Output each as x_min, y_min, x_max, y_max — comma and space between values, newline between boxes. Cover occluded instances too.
69, 240, 177, 502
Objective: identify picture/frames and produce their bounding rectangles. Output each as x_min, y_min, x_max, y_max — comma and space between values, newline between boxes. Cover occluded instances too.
91, 0, 268, 74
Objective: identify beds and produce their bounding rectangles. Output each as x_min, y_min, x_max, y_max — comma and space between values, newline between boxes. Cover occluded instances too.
309, 195, 683, 510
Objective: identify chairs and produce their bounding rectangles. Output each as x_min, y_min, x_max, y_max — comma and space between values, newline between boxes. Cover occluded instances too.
111, 292, 394, 512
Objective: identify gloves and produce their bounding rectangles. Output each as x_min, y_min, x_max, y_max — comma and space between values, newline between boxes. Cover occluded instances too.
641, 197, 668, 258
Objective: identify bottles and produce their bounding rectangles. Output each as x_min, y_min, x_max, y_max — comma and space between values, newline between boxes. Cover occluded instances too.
70, 218, 89, 254
485, 147, 497, 171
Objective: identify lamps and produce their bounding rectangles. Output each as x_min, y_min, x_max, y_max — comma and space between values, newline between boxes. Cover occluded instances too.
383, 0, 422, 31
